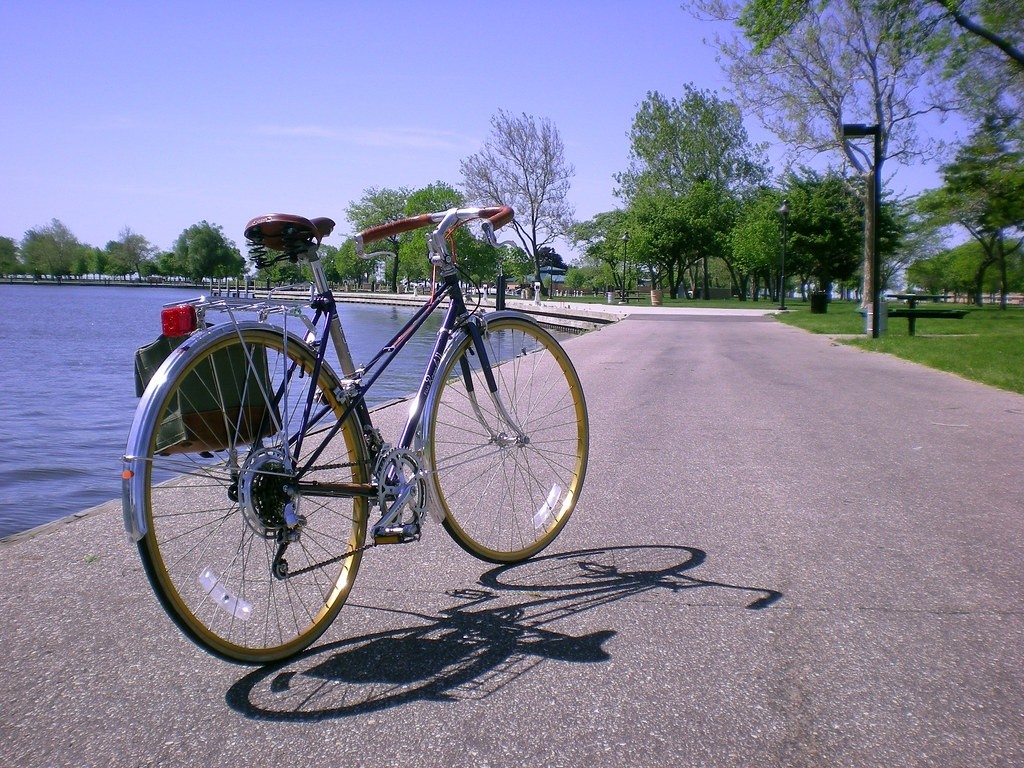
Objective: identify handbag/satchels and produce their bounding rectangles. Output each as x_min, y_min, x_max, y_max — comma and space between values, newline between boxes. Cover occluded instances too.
133, 322, 283, 456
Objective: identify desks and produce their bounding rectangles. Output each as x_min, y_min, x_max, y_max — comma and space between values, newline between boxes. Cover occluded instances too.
615, 290, 640, 302
883, 295, 956, 336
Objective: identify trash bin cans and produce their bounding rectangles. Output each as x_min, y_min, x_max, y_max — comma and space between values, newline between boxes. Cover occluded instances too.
809, 291, 828, 314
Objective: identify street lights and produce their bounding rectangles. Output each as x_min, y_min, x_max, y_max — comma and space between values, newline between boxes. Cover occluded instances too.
622, 231, 632, 302
778, 199, 793, 309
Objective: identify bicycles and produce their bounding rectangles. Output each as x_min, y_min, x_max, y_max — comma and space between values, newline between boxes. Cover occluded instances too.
121, 201, 588, 669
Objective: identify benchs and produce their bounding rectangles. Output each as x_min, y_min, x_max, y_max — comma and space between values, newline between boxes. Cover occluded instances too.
614, 297, 646, 299
856, 308, 970, 319
